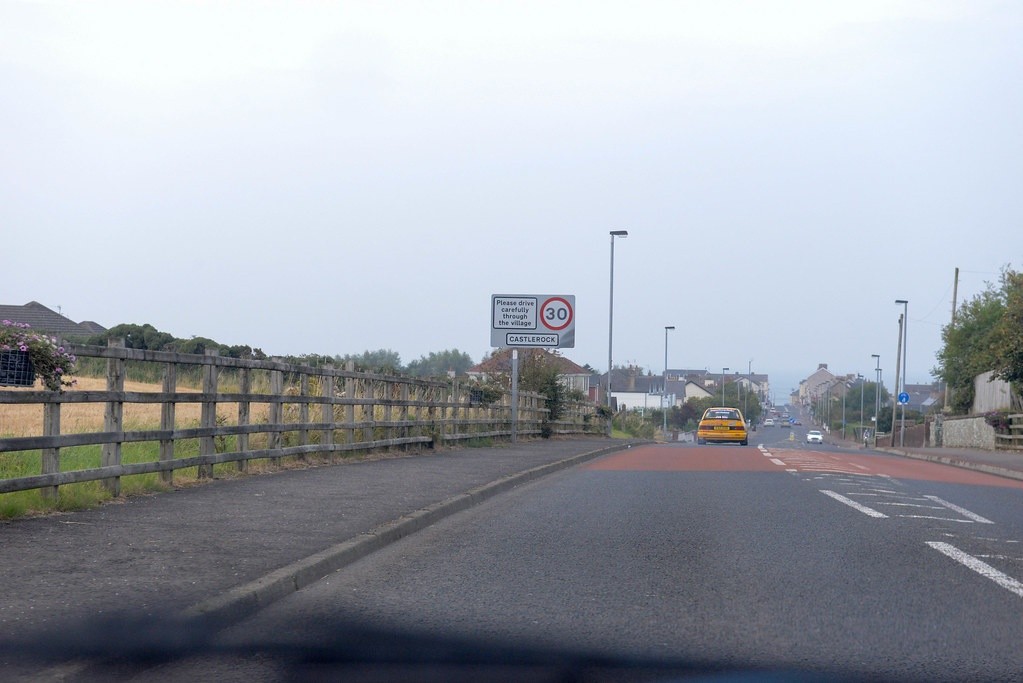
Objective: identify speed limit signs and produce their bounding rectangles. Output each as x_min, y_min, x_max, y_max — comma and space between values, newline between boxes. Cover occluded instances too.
539, 296, 573, 330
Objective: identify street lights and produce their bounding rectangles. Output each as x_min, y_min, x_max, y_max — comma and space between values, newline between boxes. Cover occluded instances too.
606, 230, 629, 434
663, 326, 676, 435
722, 368, 729, 406
871, 354, 880, 448
894, 299, 909, 447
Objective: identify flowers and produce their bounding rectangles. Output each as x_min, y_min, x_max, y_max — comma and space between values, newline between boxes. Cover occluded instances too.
0, 318, 80, 395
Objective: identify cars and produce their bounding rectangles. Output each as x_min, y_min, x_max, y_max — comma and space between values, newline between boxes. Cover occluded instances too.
764, 407, 801, 429
697, 407, 749, 446
805, 431, 824, 445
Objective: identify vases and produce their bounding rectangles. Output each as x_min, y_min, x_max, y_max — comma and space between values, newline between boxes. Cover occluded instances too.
0, 347, 37, 387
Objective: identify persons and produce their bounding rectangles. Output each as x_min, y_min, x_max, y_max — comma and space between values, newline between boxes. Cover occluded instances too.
864, 430, 869, 442
